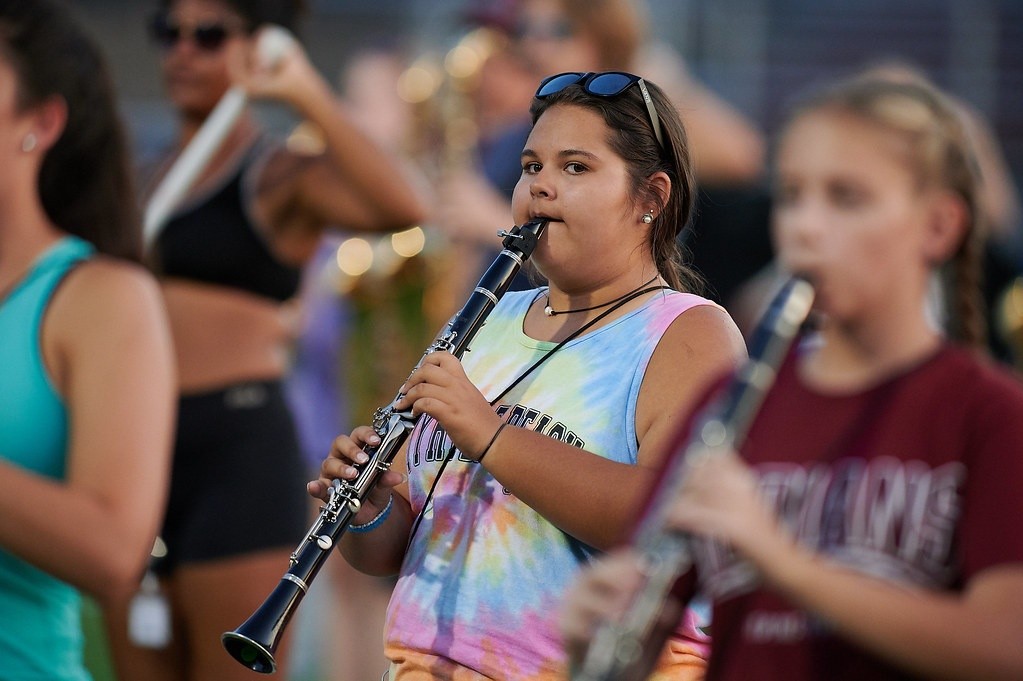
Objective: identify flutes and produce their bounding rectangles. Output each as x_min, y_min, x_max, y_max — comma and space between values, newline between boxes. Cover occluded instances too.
575, 275, 818, 681
221, 216, 548, 675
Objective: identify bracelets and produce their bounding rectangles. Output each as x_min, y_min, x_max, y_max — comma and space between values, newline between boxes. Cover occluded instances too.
478, 422, 506, 463
348, 495, 394, 533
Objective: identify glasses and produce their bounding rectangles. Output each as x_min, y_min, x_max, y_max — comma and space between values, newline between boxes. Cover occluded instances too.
535, 70, 665, 153
147, 18, 247, 50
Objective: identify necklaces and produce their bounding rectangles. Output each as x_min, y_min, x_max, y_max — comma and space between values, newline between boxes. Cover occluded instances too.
543, 273, 658, 315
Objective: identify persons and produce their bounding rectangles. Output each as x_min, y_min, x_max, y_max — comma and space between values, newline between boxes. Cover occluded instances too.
102, 0, 426, 681
334, 0, 764, 399
0, 0, 180, 681
307, 70, 750, 681
562, 67, 1023, 681
